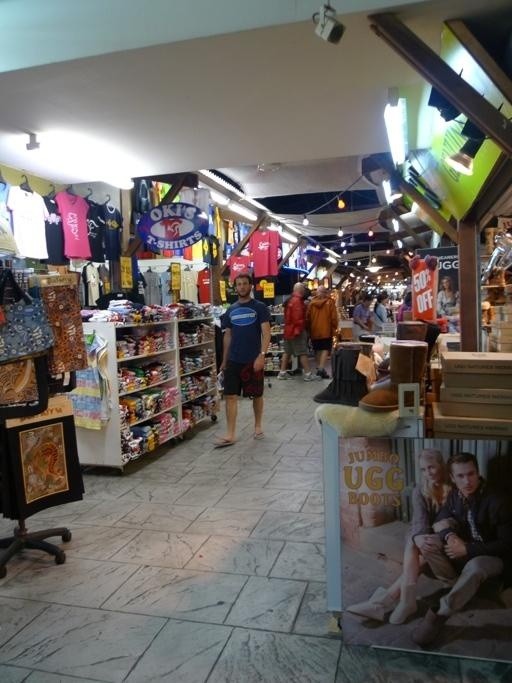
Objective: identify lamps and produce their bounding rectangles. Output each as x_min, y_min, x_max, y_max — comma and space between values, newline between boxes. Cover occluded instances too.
442, 117, 487, 177
382, 85, 410, 167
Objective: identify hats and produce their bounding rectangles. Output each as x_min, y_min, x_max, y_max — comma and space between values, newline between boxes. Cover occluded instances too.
317, 285, 325, 295
293, 282, 305, 291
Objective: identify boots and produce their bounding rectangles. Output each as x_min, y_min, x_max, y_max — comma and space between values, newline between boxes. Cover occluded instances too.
346, 583, 449, 646
313, 320, 441, 412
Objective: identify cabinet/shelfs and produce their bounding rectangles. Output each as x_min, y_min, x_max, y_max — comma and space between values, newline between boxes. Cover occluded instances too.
72, 317, 218, 475
263, 304, 300, 373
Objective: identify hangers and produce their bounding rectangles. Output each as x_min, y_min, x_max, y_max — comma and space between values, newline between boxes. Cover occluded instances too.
0, 174, 115, 208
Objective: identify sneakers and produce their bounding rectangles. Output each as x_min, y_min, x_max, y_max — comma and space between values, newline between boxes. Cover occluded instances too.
277, 366, 331, 382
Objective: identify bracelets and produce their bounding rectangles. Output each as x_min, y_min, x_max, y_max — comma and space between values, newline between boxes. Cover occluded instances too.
260, 349, 267, 355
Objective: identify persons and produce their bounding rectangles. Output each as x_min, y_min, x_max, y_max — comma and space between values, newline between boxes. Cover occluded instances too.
276, 282, 412, 381
436, 276, 460, 319
212, 275, 272, 449
410, 451, 512, 651
346, 448, 458, 625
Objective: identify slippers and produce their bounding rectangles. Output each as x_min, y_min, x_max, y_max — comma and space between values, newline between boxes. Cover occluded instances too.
211, 438, 232, 445
254, 432, 264, 439
